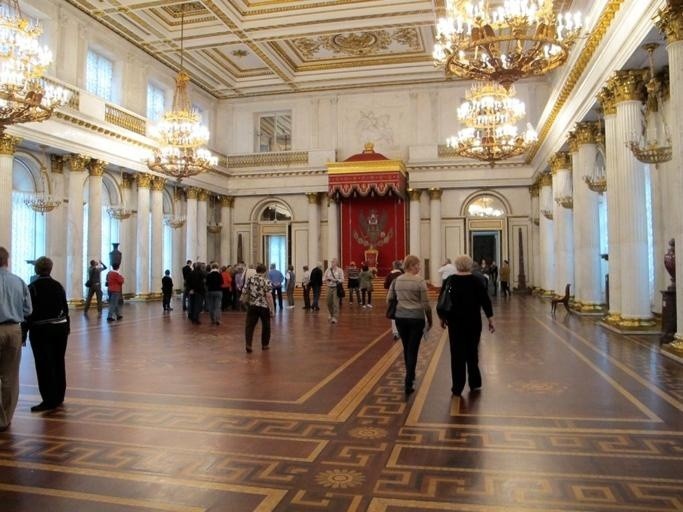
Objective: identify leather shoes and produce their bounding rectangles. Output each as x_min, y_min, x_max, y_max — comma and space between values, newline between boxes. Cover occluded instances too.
31, 400, 55, 411
408, 384, 416, 396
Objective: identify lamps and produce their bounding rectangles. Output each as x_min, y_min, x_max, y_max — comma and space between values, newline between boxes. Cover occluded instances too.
616, 123, 674, 166
22, 170, 61, 216
581, 163, 612, 195
0, 0, 72, 129
163, 213, 185, 228
530, 193, 573, 225
430, 0, 588, 88
143, 0, 216, 182
106, 206, 135, 220
436, 80, 540, 165
206, 217, 222, 234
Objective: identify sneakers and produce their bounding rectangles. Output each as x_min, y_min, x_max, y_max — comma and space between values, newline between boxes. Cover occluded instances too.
350, 302, 373, 308
246, 345, 252, 351
472, 386, 482, 391
262, 345, 271, 350
328, 314, 338, 323
117, 316, 122, 320
286, 305, 295, 309
216, 320, 223, 325
107, 317, 115, 321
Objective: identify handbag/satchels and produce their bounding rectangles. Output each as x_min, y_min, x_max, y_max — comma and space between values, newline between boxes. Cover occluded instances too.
85, 280, 92, 287
336, 282, 346, 297
437, 281, 453, 321
239, 289, 249, 303
386, 298, 398, 319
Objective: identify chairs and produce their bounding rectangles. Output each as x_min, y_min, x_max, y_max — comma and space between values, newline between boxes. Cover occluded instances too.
359, 245, 378, 278
550, 282, 572, 315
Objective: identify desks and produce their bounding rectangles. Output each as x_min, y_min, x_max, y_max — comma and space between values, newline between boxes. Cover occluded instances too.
659, 288, 676, 347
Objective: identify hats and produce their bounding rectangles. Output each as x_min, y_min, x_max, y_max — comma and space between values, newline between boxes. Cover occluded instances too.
27, 257, 53, 270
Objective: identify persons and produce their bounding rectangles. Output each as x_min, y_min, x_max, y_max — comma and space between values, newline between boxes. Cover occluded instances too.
105, 262, 124, 321
182, 259, 256, 325
438, 259, 457, 282
384, 254, 433, 398
22, 256, 70, 413
161, 270, 173, 311
240, 264, 275, 352
436, 254, 496, 396
384, 262, 402, 340
0, 247, 34, 430
83, 260, 108, 314
474, 261, 512, 297
268, 259, 377, 324
